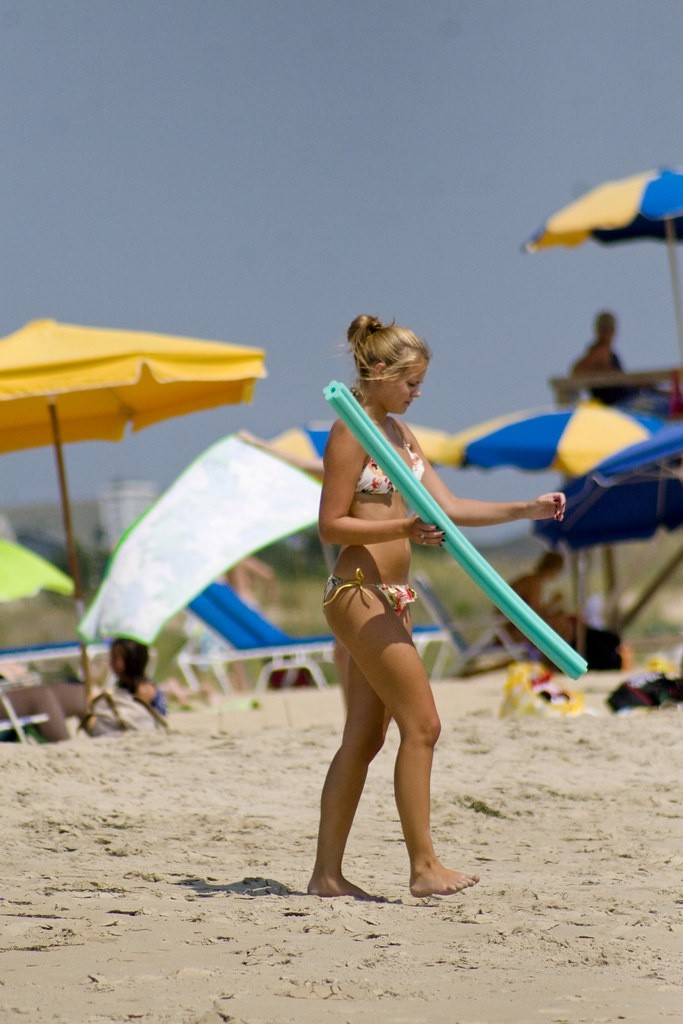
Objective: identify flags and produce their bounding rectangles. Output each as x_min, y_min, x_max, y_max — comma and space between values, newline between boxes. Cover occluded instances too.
76, 431, 321, 643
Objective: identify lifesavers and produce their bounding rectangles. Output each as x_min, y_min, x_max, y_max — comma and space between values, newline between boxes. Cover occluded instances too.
323, 379, 590, 682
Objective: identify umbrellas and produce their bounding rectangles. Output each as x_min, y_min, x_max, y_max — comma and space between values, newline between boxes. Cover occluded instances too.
268, 402, 683, 666
1, 314, 265, 650
0, 540, 73, 606
518, 164, 683, 257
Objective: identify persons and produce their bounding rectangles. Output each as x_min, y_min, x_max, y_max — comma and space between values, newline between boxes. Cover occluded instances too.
569, 309, 625, 401
495, 551, 581, 663
306, 314, 483, 899
106, 639, 167, 739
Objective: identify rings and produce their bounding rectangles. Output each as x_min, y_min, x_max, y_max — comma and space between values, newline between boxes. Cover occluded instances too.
419, 532, 425, 538
422, 540, 426, 544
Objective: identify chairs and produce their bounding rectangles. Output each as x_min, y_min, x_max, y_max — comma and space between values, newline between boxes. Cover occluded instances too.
177, 575, 542, 710
1, 639, 158, 743
